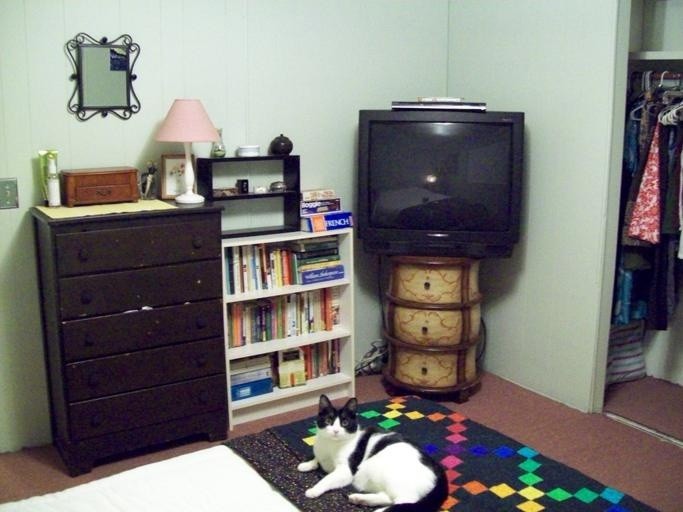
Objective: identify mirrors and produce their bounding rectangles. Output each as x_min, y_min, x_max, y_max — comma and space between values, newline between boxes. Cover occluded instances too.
65, 32, 140, 120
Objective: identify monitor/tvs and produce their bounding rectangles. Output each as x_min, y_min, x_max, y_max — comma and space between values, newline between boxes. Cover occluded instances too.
359, 112, 523, 259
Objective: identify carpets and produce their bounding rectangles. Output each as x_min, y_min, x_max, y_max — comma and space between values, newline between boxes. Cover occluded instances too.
223, 394, 662, 511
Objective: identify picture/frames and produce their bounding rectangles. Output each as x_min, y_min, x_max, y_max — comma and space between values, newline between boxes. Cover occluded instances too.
160, 153, 196, 199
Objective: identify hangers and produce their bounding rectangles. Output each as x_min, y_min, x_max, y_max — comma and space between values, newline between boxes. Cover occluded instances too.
628, 71, 683, 127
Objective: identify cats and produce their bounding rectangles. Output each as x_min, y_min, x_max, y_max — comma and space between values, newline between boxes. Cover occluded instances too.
296, 393, 448, 512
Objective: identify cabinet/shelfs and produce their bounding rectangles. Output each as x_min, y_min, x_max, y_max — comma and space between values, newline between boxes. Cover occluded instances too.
30, 206, 230, 476
197, 155, 302, 240
382, 257, 483, 403
221, 227, 356, 430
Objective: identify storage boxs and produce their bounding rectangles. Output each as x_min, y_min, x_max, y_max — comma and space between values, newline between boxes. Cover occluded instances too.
62, 167, 140, 208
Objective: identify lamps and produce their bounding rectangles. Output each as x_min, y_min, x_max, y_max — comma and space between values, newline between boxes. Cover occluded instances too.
154, 99, 220, 205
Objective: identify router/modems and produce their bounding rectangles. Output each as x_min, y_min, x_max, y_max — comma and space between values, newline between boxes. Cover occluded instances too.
392, 101, 488, 111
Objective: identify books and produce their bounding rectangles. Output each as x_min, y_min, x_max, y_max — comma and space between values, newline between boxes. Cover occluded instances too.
224, 189, 355, 401
